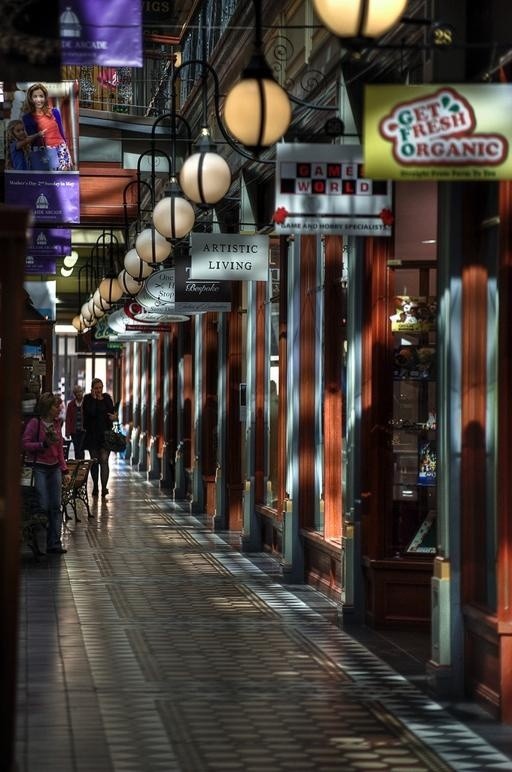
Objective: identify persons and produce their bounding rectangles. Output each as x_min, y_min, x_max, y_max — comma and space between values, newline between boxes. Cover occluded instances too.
22, 392, 68, 555
66, 385, 87, 460
82, 377, 117, 496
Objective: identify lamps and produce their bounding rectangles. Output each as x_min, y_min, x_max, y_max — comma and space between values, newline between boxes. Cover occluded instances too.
72, 0, 471, 352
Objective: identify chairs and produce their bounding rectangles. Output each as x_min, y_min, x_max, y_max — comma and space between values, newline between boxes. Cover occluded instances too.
61, 458, 97, 523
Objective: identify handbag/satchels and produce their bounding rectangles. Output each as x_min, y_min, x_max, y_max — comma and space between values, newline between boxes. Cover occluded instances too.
100, 412, 127, 452
19, 417, 41, 488
